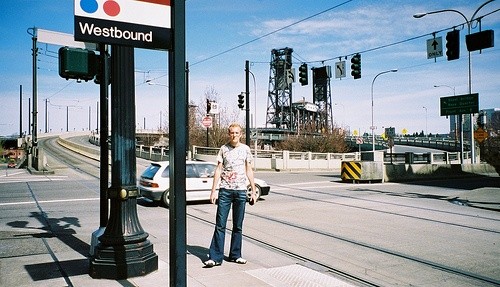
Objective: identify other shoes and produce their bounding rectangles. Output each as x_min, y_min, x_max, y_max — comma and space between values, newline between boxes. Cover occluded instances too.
228, 257, 247, 264
204, 260, 221, 267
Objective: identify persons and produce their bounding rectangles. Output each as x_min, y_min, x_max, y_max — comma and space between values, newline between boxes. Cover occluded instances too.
204, 123, 256, 268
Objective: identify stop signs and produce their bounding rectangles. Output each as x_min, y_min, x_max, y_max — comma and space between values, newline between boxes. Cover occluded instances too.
202, 116, 212, 128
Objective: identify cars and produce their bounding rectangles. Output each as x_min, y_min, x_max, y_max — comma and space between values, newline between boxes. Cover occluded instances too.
139, 161, 270, 208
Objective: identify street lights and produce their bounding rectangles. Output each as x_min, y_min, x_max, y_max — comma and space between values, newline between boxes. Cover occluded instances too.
370, 68, 398, 150
434, 84, 458, 145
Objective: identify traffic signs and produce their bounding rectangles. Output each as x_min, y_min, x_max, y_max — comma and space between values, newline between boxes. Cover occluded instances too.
426, 36, 443, 58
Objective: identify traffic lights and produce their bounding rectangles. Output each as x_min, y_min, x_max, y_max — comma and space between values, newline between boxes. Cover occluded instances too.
237, 94, 245, 108
350, 54, 362, 79
446, 30, 460, 61
298, 63, 308, 86
